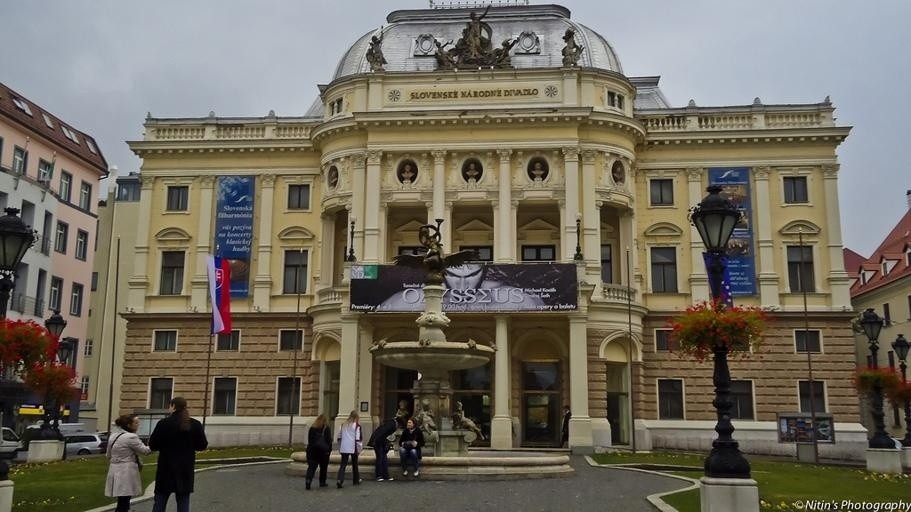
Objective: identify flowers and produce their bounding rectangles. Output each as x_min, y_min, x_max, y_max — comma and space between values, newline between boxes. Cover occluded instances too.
851, 368, 901, 400
668, 303, 768, 363
53, 386, 76, 399
23, 362, 79, 395
887, 380, 911, 406
0, 320, 58, 375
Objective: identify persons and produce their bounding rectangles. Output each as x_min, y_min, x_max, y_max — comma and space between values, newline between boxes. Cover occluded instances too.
305, 400, 426, 490
561, 27, 584, 68
104, 413, 151, 512
560, 405, 570, 448
433, 4, 517, 72
375, 260, 550, 311
366, 25, 388, 73
414, 398, 439, 435
453, 400, 485, 440
150, 396, 208, 512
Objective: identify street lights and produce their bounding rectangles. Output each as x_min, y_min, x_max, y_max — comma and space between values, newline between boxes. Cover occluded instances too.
51, 337, 72, 442
573, 211, 587, 260
346, 214, 360, 263
35, 309, 68, 439
858, 305, 905, 477
686, 184, 763, 512
0, 206, 40, 320
889, 331, 911, 470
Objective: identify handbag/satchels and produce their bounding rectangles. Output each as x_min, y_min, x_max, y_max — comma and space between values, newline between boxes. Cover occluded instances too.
317, 436, 330, 455
384, 439, 394, 454
355, 441, 362, 454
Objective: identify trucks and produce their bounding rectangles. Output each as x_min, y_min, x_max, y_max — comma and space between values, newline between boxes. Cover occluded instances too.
1, 427, 26, 458
26, 418, 87, 434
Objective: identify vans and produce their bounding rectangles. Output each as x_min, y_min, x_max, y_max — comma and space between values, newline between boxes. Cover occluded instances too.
60, 431, 109, 455
